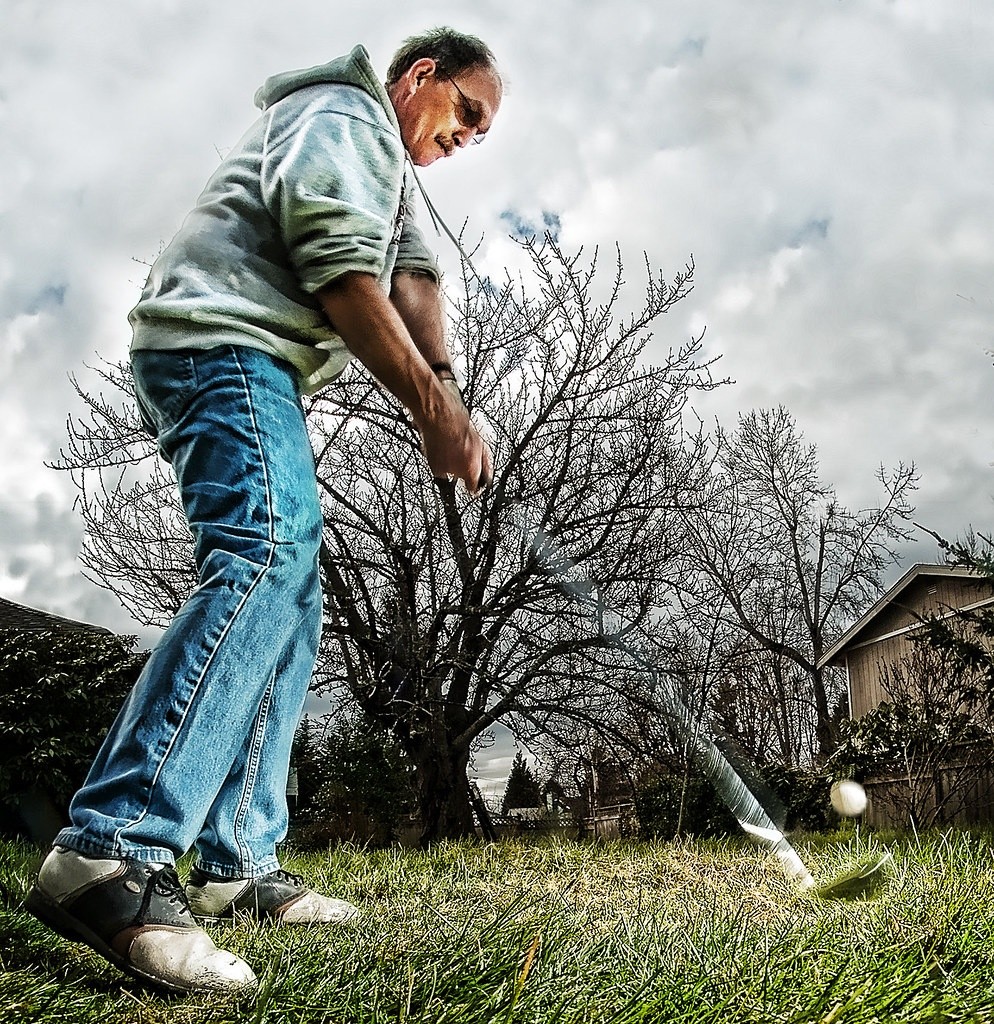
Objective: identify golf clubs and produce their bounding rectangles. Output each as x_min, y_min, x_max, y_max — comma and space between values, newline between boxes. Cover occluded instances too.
486, 482, 889, 903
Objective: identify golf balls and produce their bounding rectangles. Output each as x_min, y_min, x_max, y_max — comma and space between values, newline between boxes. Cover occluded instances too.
827, 783, 868, 816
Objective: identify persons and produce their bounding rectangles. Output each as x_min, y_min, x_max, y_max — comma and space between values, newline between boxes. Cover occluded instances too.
21, 24, 504, 995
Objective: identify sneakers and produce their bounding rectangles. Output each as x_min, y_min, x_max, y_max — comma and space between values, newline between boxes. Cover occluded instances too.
184, 864, 361, 925
23, 848, 259, 996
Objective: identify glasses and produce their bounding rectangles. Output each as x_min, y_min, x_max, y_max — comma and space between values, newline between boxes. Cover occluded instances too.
437, 64, 484, 146
